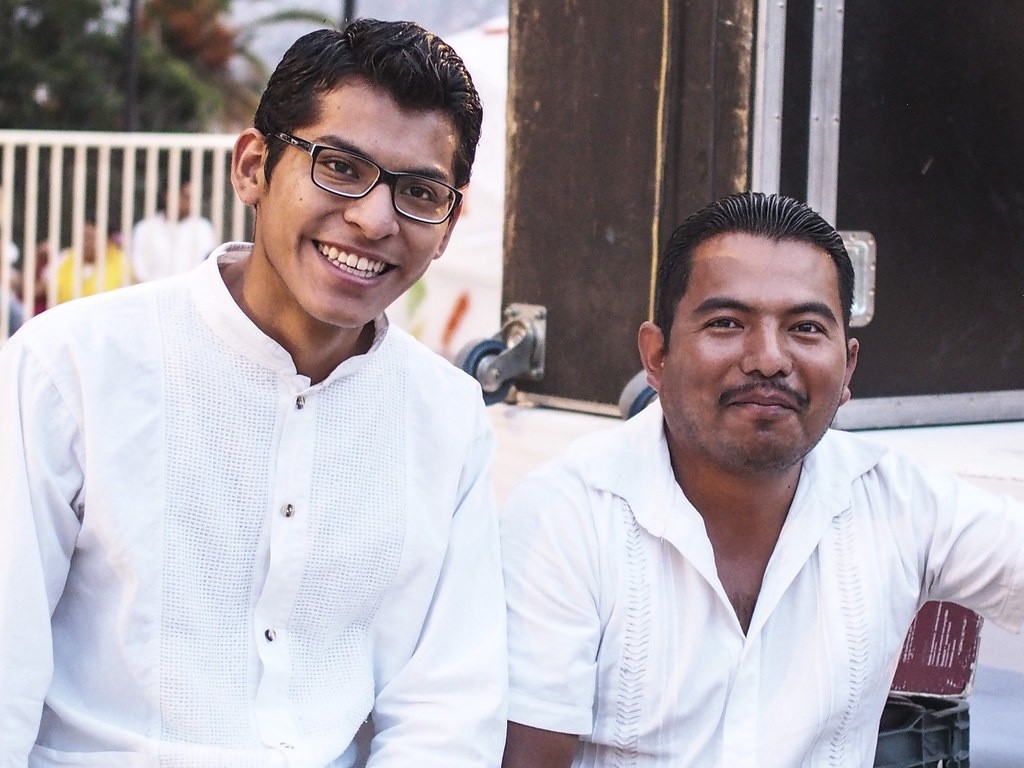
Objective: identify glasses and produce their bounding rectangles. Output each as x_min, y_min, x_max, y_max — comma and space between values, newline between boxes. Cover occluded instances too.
268, 132, 462, 224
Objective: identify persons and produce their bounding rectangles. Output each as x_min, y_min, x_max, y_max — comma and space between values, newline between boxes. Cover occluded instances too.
478, 191, 1024, 768
0, 172, 216, 339
0, 19, 506, 768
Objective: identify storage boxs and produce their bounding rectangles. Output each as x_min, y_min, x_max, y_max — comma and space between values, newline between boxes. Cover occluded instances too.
871, 690, 970, 768
